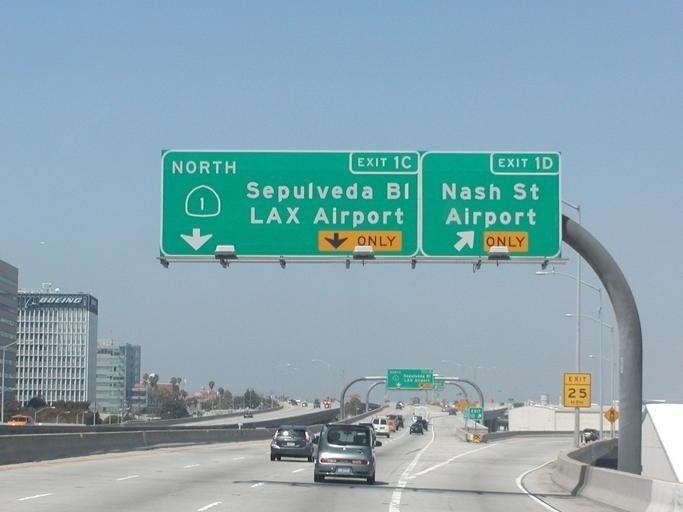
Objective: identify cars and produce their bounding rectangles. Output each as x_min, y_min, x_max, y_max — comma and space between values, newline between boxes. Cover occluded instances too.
269, 396, 457, 484
243, 410, 252, 418
5, 415, 34, 425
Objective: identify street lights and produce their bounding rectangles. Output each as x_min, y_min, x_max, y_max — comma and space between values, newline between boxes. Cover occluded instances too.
535, 269, 616, 440
55, 410, 70, 425
0, 339, 33, 423
34, 406, 55, 424
76, 411, 88, 424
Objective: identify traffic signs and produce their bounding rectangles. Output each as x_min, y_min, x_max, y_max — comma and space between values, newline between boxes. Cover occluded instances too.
432, 379, 444, 391
469, 408, 482, 419
158, 152, 420, 260
386, 368, 433, 389
418, 152, 560, 260
